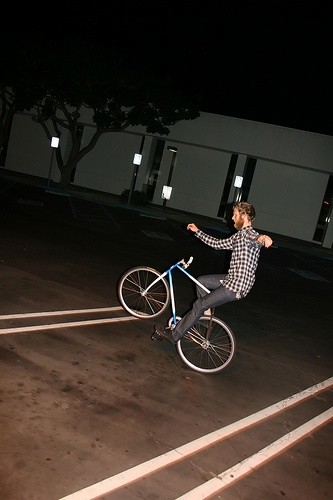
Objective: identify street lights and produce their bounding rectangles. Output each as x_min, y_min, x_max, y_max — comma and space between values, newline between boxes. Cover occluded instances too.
127, 153, 143, 204
47, 136, 60, 186
160, 145, 178, 209
233, 175, 244, 202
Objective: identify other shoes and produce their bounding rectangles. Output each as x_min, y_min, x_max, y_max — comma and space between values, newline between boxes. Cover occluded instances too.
198, 316, 213, 329
155, 323, 172, 341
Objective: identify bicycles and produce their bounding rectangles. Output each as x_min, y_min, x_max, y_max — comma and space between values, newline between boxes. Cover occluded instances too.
118, 256, 237, 374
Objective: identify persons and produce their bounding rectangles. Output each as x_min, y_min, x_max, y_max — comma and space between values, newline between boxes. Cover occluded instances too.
155, 201, 273, 345
